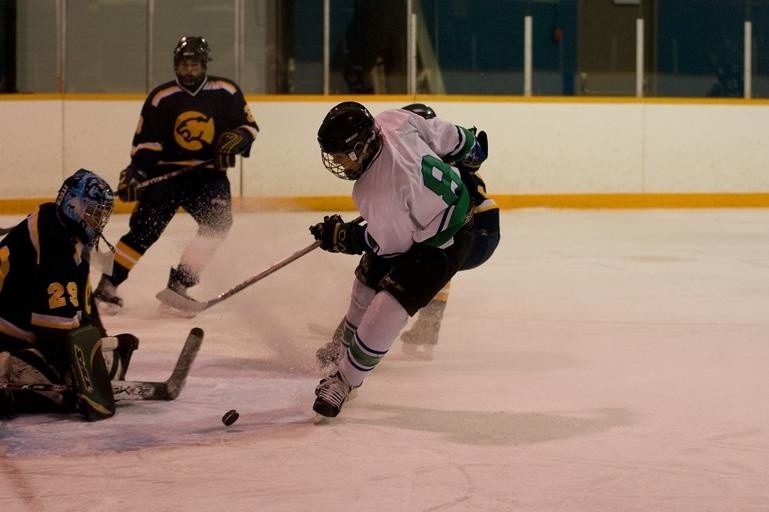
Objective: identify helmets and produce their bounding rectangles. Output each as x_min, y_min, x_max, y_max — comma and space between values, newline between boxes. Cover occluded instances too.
55, 168, 113, 245
172, 36, 214, 63
319, 102, 383, 180
401, 103, 437, 119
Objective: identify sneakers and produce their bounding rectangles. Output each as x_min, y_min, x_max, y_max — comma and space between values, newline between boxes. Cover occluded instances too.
313, 369, 352, 417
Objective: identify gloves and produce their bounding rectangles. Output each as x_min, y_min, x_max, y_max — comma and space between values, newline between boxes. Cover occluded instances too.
310, 214, 363, 255
117, 164, 148, 201
213, 130, 248, 170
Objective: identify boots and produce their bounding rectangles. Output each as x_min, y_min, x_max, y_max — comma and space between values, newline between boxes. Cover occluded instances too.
93, 273, 123, 305
167, 263, 199, 302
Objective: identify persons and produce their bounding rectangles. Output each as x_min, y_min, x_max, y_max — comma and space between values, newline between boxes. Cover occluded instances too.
398, 104, 499, 361
91, 38, 259, 319
307, 101, 476, 426
0, 168, 139, 422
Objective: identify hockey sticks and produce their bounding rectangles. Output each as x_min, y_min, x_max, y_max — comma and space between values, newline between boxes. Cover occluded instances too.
156, 216, 364, 313
0, 328, 204, 399
